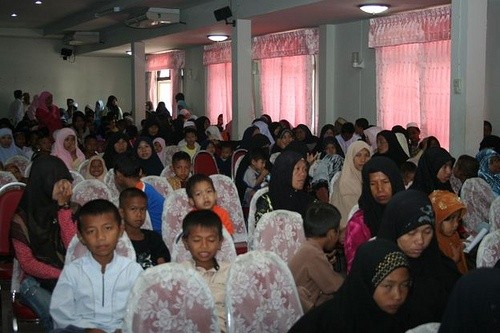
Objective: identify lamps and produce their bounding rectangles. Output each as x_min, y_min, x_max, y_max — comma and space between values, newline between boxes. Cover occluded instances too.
359, 4, 389, 14
207, 34, 229, 42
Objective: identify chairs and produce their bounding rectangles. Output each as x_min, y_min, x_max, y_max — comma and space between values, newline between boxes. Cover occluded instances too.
0, 147, 273, 333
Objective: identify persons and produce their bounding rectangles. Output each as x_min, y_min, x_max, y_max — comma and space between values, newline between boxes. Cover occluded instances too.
0, 89, 500, 333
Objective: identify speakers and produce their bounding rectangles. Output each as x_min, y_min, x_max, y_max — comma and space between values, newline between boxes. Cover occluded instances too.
61, 47, 73, 56
213, 6, 233, 22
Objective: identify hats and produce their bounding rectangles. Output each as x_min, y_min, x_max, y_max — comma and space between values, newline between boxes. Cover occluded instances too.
406, 122, 418, 128
336, 117, 347, 126
